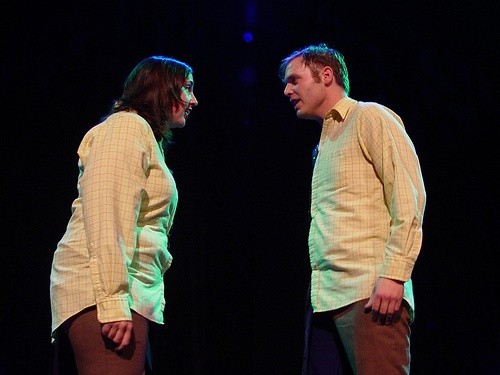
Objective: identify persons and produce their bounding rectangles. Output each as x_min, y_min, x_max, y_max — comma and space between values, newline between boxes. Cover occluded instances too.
279, 44, 426, 375
50, 56, 199, 375
